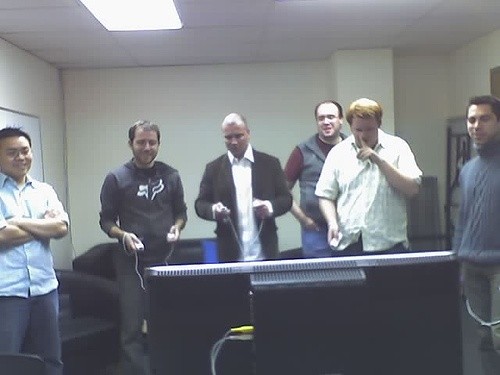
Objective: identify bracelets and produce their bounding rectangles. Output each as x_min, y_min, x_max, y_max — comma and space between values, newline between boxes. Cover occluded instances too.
122, 233, 132, 254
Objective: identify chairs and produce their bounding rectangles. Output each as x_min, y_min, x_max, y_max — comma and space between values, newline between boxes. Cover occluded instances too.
73, 243, 118, 278
56, 269, 119, 374
0, 352, 45, 374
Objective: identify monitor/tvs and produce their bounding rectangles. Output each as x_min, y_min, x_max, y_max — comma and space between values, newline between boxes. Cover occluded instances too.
142, 250, 463, 373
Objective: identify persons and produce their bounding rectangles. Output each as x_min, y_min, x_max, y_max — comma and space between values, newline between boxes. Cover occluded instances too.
0, 127, 70, 375
448, 97, 500, 374
283, 99, 369, 258
193, 112, 295, 261
314, 97, 423, 257
99, 122, 189, 374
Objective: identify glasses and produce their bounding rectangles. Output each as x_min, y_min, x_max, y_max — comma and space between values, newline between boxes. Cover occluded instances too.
225, 132, 244, 140
